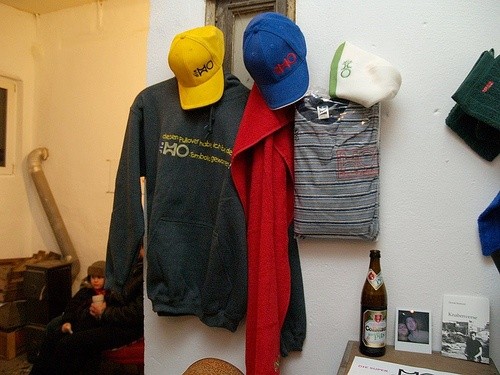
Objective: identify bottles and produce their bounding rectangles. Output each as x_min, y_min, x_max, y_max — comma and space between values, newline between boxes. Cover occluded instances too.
359, 252, 388, 358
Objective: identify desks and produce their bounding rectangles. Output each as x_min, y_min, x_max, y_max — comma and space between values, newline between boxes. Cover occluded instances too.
336, 340, 500, 375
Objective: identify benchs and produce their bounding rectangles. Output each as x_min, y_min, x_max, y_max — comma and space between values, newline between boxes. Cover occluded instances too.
101, 339, 144, 375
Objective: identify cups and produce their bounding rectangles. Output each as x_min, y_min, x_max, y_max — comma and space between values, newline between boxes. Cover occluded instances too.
92, 295, 104, 304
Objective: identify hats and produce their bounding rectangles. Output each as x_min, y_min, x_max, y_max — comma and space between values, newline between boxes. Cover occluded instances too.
168, 25, 225, 110
87, 260, 107, 277
243, 12, 310, 110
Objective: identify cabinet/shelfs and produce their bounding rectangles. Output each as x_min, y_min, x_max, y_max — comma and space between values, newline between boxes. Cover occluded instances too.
22, 260, 74, 364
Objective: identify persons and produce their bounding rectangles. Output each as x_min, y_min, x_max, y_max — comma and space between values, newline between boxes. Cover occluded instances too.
55, 243, 144, 375
33, 261, 111, 375
477, 328, 490, 358
451, 323, 466, 343
398, 317, 411, 342
405, 315, 429, 344
464, 331, 483, 362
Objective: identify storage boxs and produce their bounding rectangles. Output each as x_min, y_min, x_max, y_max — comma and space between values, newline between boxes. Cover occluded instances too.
0, 257, 33, 360
